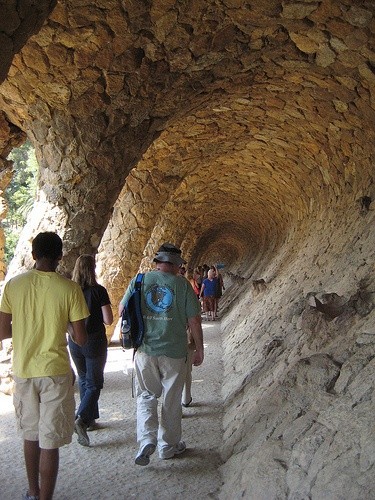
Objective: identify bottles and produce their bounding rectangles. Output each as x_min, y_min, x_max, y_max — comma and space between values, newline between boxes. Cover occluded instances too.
122, 320, 131, 349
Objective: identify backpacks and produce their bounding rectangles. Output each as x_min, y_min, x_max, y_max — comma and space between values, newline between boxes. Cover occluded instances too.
120, 273, 145, 348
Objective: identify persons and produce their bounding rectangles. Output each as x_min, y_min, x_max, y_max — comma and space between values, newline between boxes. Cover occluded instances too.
119, 244, 205, 466
178, 319, 194, 407
0, 231, 90, 500
182, 265, 224, 322
66, 255, 113, 447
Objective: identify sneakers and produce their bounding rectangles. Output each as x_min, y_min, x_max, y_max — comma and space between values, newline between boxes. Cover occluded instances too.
135, 442, 156, 466
73, 415, 90, 446
86, 420, 105, 430
159, 441, 186, 459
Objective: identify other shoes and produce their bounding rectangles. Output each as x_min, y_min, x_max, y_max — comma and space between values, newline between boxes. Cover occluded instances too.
182, 398, 192, 406
21, 487, 39, 500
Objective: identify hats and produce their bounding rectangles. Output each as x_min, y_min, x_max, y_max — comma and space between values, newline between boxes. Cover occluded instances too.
153, 243, 187, 264
